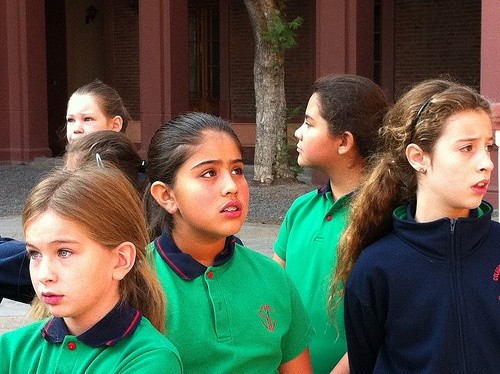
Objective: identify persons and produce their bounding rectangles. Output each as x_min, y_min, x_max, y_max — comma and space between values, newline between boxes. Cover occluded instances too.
325, 79, 500, 374
0, 130, 154, 307
1, 161, 183, 374
65, 81, 132, 144
272, 74, 389, 374
142, 112, 315, 374
